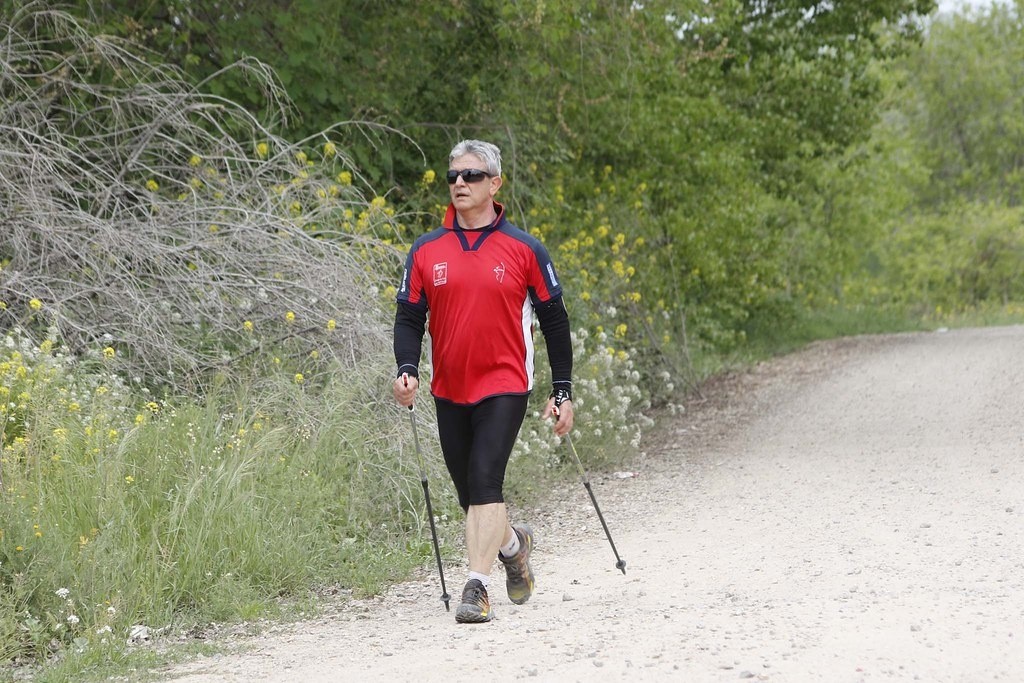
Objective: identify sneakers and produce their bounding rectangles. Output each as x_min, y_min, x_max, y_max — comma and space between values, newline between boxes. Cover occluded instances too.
455, 579, 495, 623
498, 526, 535, 604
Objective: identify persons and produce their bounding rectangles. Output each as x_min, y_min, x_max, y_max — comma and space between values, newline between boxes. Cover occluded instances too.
394, 140, 575, 624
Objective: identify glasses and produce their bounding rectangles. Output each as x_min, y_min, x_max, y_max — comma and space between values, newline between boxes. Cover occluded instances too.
446, 168, 491, 184
